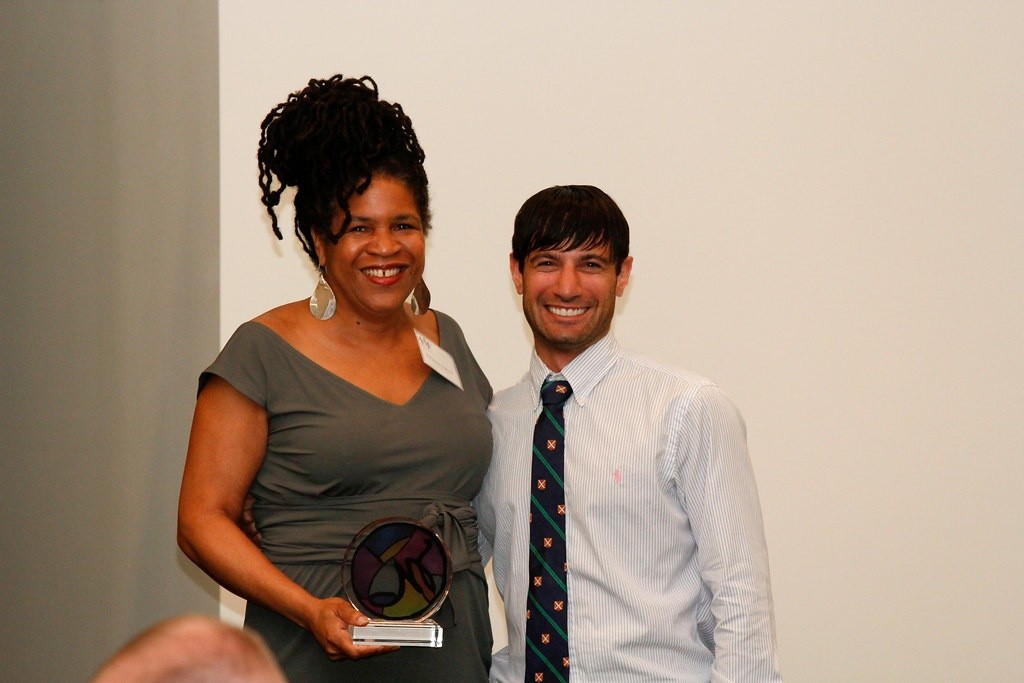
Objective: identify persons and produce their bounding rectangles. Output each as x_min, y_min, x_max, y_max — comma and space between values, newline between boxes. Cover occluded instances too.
471, 186, 780, 683
90, 612, 287, 683
177, 73, 494, 683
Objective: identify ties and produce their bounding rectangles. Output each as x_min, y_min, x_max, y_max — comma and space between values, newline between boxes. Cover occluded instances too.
524, 379, 573, 683
